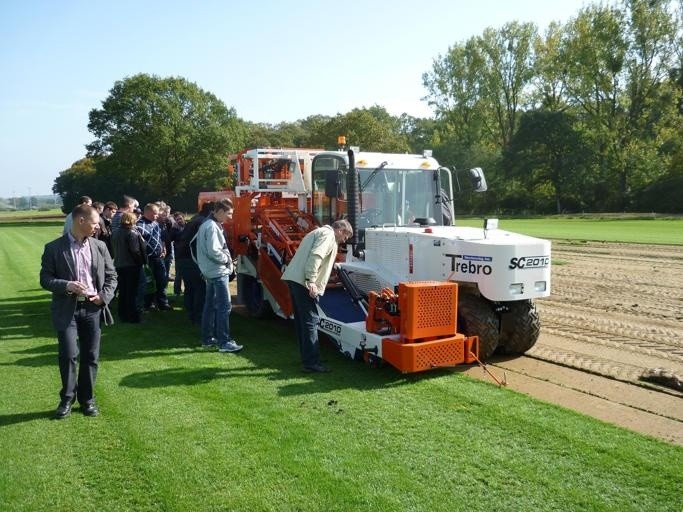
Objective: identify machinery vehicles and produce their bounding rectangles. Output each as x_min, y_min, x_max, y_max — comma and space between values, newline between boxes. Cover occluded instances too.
198, 136, 551, 387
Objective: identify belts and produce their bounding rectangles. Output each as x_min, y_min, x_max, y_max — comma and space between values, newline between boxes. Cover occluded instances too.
77, 297, 88, 302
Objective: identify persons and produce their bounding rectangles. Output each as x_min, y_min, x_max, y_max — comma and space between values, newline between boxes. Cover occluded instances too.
39, 202, 118, 419
397, 200, 416, 226
281, 218, 352, 374
60, 194, 244, 354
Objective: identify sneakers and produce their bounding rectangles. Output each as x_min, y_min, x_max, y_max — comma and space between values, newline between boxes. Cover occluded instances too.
200, 337, 218, 348
120, 292, 205, 325
217, 339, 243, 353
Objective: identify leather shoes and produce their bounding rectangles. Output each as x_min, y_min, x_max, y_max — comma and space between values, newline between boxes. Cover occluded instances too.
301, 359, 332, 375
79, 403, 98, 417
54, 397, 74, 418
